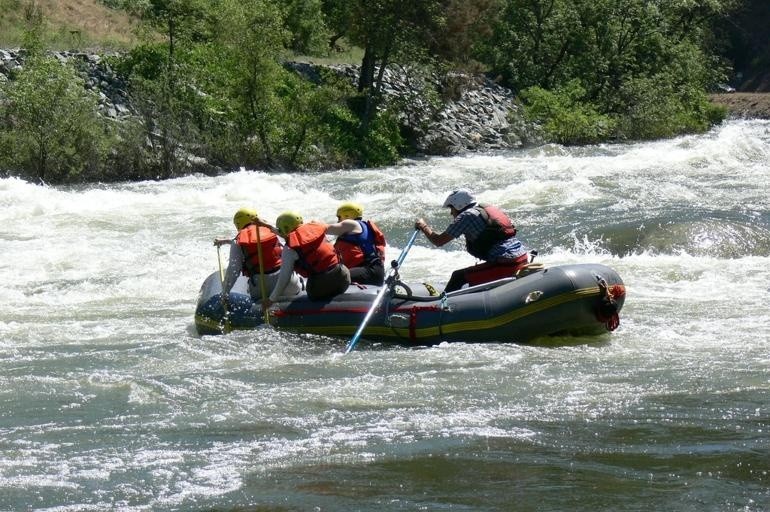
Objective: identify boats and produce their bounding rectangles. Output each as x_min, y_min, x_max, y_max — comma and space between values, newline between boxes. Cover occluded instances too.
194, 262, 623, 344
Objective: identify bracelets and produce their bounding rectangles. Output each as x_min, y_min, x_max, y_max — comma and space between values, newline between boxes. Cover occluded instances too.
421, 225, 428, 230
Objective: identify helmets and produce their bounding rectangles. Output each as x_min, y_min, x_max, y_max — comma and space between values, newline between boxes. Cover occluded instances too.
336, 203, 363, 222
442, 189, 478, 210
233, 207, 258, 231
276, 211, 304, 237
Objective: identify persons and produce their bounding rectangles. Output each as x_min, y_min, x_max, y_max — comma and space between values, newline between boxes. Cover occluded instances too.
264, 211, 351, 306
214, 207, 305, 299
319, 202, 385, 286
415, 188, 528, 292
